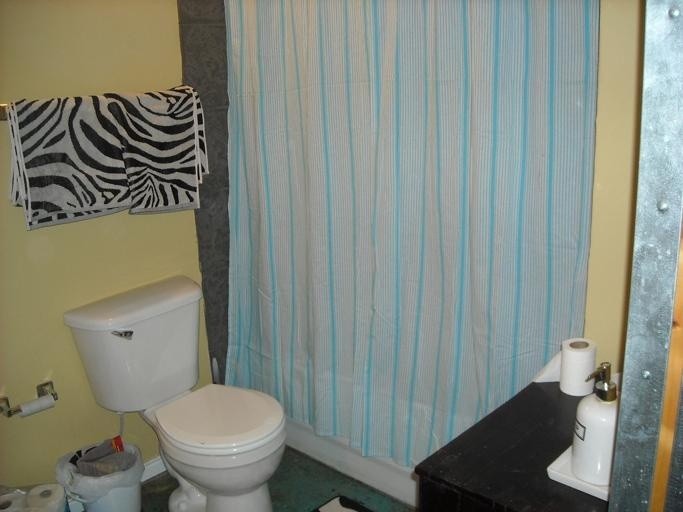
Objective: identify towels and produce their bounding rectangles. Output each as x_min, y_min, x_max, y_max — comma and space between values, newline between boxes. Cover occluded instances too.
7, 83, 208, 232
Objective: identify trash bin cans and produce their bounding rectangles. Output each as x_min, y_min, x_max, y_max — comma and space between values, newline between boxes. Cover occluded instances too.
56, 440, 144, 511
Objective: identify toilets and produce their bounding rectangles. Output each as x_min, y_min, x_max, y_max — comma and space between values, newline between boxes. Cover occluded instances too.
64, 274, 286, 512
